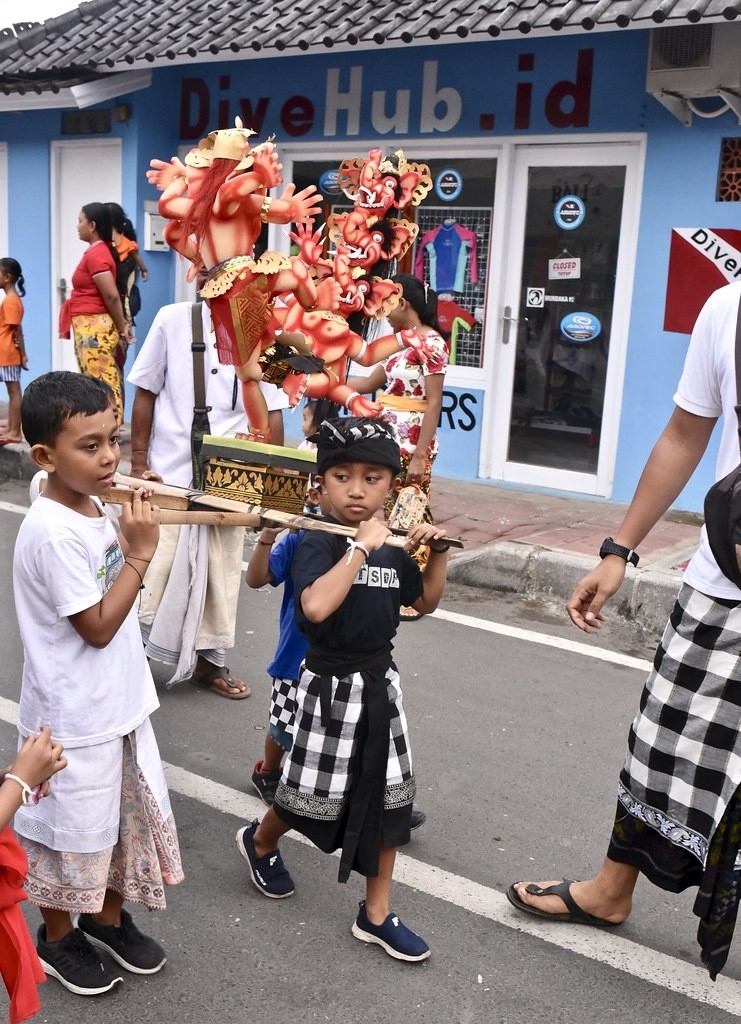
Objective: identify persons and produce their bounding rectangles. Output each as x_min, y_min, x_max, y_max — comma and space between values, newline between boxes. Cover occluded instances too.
127, 300, 292, 699
110, 208, 148, 282
59, 202, 137, 445
0, 257, 30, 444
505, 281, 741, 982
297, 399, 339, 451
236, 418, 432, 963
343, 274, 449, 621
0, 725, 68, 1023
104, 202, 139, 430
13, 371, 186, 996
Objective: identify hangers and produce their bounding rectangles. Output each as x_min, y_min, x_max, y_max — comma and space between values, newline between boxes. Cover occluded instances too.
442, 212, 457, 227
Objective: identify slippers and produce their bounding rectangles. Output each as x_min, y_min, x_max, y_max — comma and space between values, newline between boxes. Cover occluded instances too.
0, 436, 20, 444
506, 878, 619, 925
197, 666, 250, 700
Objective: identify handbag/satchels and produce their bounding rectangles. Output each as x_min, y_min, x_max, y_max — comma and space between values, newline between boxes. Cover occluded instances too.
702, 467, 741, 588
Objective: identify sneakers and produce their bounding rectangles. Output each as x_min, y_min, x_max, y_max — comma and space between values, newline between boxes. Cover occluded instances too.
236, 820, 295, 899
350, 900, 430, 962
409, 810, 426, 831
72, 907, 166, 975
35, 920, 123, 996
251, 759, 284, 805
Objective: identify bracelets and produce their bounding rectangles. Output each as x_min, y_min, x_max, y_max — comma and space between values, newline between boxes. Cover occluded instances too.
348, 546, 367, 560
345, 536, 369, 566
430, 545, 450, 553
141, 269, 147, 272
126, 556, 151, 563
124, 561, 145, 614
4, 773, 41, 806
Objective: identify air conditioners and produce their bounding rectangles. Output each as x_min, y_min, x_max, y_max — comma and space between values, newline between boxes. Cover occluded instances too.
645, 23, 741, 98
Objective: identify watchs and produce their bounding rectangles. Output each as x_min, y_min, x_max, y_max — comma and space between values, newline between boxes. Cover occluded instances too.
599, 537, 640, 567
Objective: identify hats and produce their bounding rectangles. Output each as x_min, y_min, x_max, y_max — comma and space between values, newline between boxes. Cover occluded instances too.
305, 415, 401, 478
